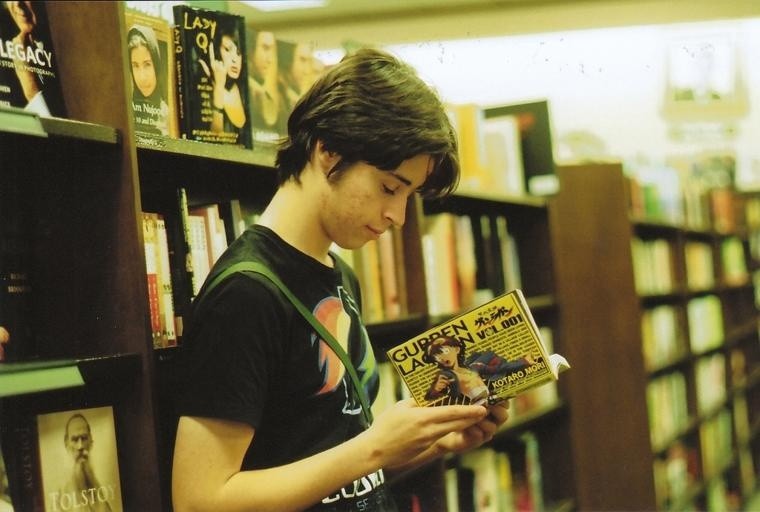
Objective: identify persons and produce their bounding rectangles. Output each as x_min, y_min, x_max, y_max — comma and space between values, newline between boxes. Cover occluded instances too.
168, 47, 508, 512
0, 1, 55, 118
198, 26, 246, 146
248, 28, 319, 145
127, 23, 167, 136
44, 413, 112, 511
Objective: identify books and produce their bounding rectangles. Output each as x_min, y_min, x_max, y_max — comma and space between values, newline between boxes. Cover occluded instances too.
623, 175, 759, 512
372, 326, 559, 425
172, 3, 253, 150
386, 290, 570, 409
141, 186, 522, 350
122, 8, 173, 138
442, 97, 559, 206
397, 429, 545, 512
